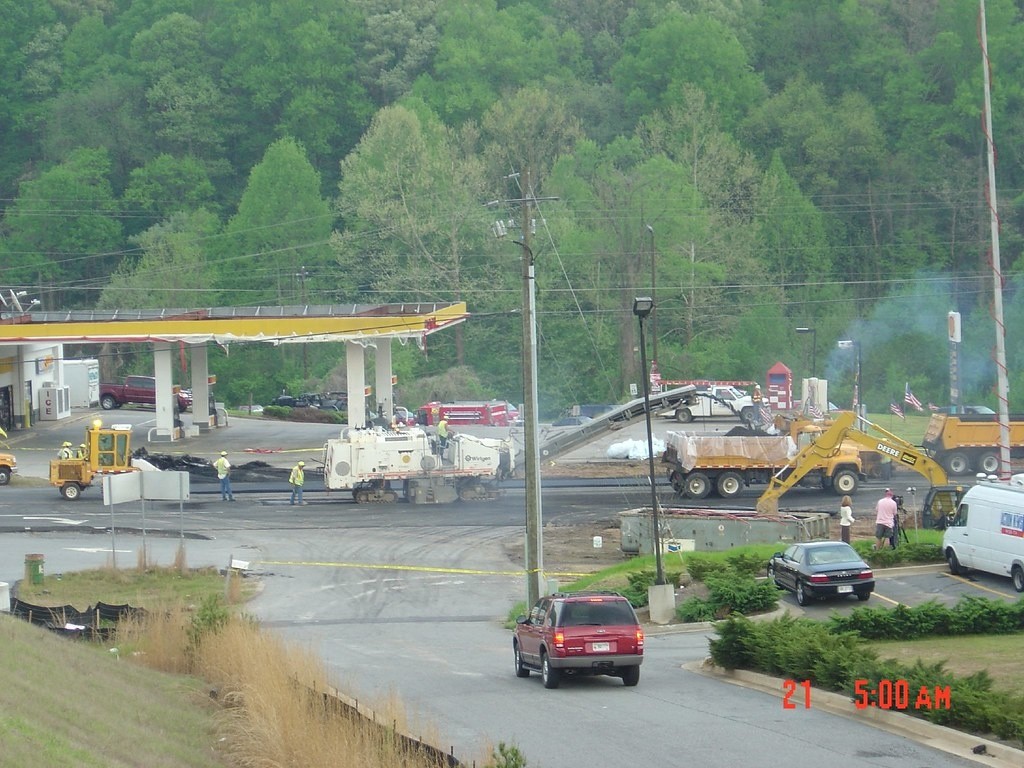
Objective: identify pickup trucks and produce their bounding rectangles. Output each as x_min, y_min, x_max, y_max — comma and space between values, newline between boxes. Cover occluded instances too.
99, 376, 192, 412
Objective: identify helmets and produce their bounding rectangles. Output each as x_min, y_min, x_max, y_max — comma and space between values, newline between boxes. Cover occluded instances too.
80, 444, 86, 448
221, 451, 227, 455
62, 442, 67, 447
67, 442, 72, 446
298, 461, 305, 467
444, 416, 449, 419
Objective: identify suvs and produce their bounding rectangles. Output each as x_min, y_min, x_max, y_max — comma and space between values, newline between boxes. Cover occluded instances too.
512, 591, 645, 689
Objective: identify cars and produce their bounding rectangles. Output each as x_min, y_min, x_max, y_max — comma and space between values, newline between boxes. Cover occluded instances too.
766, 539, 876, 606
965, 406, 997, 415
552, 415, 592, 427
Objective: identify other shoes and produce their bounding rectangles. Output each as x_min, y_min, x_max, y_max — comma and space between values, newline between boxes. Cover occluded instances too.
222, 498, 228, 501
229, 499, 237, 501
301, 502, 307, 505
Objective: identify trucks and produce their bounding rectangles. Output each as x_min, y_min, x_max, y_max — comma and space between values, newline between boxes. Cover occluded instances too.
268, 391, 519, 426
656, 384, 770, 422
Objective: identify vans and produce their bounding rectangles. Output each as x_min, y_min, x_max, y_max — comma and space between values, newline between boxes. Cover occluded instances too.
943, 473, 1024, 593
570, 403, 624, 421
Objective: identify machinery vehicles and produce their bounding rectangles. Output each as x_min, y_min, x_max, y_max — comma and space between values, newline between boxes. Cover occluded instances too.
756, 409, 958, 525
320, 384, 698, 506
48, 419, 160, 501
0, 454, 20, 485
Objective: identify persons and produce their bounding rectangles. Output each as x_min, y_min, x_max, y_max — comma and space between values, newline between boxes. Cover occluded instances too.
99, 436, 108, 465
751, 386, 760, 422
76, 444, 86, 458
213, 452, 236, 501
58, 443, 73, 459
438, 417, 455, 456
875, 492, 897, 549
289, 462, 306, 505
841, 495, 855, 544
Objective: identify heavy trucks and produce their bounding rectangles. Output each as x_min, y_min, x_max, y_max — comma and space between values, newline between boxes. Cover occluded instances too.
658, 412, 894, 498
921, 410, 1024, 475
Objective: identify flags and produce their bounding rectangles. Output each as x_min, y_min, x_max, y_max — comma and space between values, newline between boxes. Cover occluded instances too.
905, 386, 923, 410
890, 400, 904, 419
810, 399, 822, 418
759, 403, 773, 425
929, 401, 939, 410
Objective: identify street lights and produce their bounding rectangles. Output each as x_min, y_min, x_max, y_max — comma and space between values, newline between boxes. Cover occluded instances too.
631, 296, 668, 587
838, 340, 864, 431
794, 325, 818, 377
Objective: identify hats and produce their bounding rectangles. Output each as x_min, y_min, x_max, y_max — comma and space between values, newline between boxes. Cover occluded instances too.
886, 491, 894, 497
